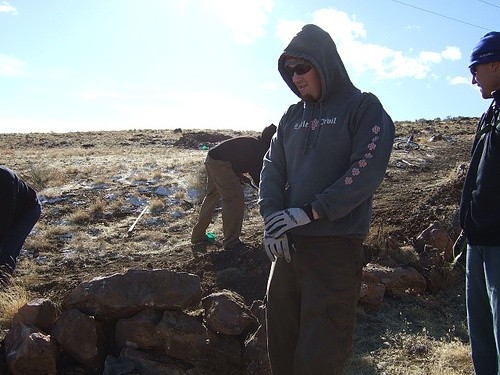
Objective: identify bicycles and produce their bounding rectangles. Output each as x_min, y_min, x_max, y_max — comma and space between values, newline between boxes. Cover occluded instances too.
393, 131, 420, 153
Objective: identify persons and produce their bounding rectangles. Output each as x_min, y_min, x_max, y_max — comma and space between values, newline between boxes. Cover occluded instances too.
460, 29, 500, 375
191, 123, 277, 249
0, 164, 42, 291
259, 24, 396, 375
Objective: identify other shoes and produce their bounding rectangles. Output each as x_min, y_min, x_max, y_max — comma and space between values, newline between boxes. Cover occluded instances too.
225, 242, 253, 256
190, 238, 218, 246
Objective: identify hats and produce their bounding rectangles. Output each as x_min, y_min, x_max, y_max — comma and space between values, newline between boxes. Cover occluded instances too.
262, 124, 277, 142
468, 31, 500, 68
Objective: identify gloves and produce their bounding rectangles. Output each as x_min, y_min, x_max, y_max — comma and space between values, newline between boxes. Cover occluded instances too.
264, 231, 296, 263
265, 204, 314, 239
453, 229, 467, 269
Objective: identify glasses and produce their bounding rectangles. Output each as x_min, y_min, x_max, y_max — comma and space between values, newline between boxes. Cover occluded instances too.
284, 64, 312, 78
